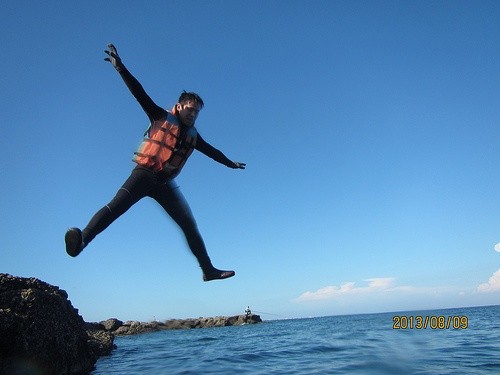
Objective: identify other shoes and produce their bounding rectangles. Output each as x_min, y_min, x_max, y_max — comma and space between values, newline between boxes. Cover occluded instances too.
203, 269, 235, 281
65, 228, 83, 257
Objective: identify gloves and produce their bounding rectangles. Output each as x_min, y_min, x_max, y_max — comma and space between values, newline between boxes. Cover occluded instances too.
232, 162, 246, 169
103, 43, 124, 71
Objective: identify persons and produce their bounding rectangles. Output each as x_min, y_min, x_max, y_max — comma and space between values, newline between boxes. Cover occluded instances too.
65, 42, 247, 281
